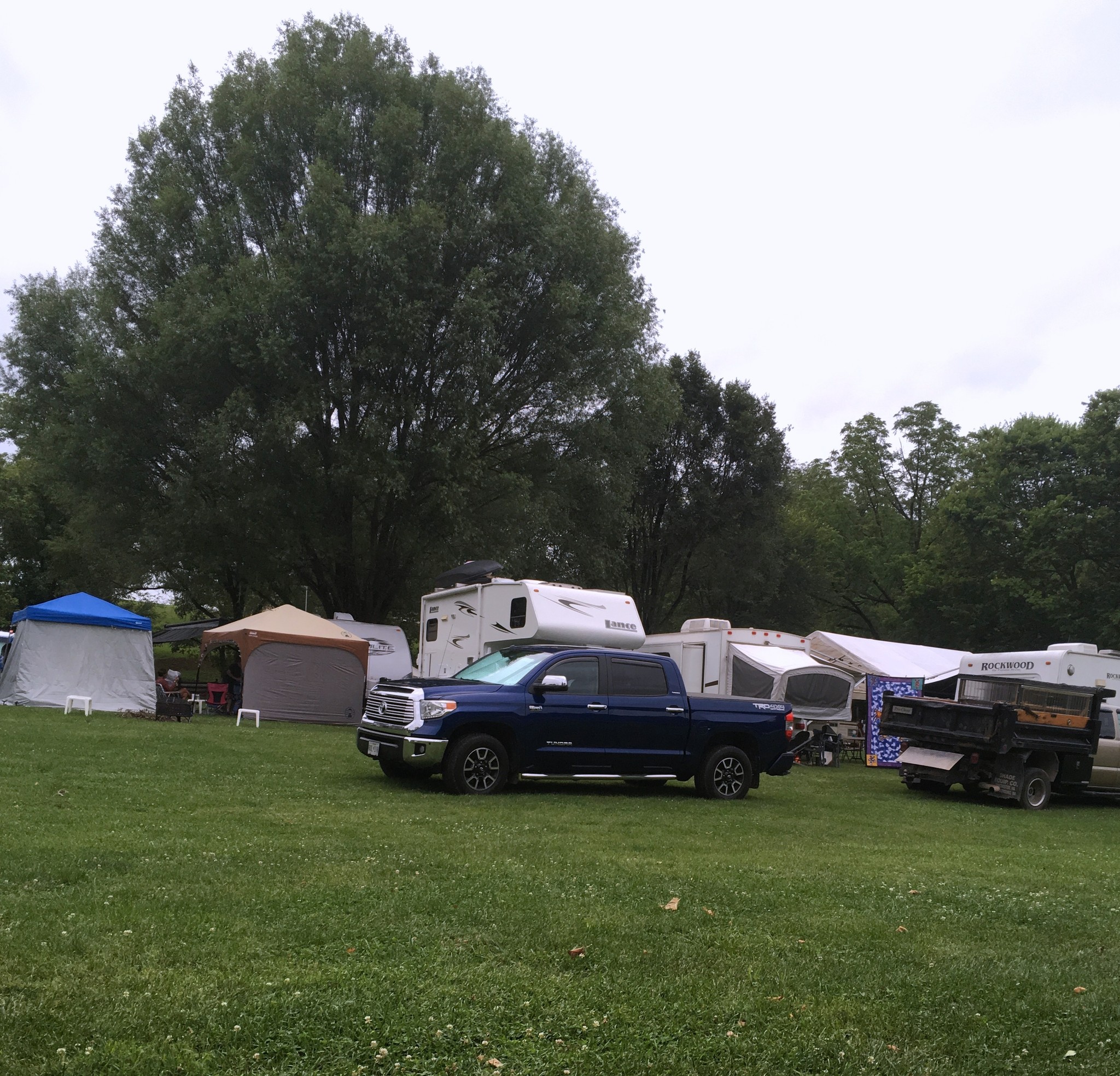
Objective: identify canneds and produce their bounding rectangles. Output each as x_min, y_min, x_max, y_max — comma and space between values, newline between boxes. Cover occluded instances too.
195, 694, 200, 700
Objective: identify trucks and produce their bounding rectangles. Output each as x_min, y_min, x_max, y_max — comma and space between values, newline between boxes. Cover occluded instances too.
623, 617, 811, 695
415, 578, 645, 678
953, 643, 1120, 798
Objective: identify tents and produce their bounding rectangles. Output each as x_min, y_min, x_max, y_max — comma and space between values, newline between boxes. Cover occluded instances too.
0, 591, 157, 714
191, 604, 371, 727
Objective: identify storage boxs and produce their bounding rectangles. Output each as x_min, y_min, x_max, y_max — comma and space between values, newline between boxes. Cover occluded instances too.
434, 559, 504, 584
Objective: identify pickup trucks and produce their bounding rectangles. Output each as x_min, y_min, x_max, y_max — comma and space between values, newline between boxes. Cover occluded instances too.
356, 645, 797, 799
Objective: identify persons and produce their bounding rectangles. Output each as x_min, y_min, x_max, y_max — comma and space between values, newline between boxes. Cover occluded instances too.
222, 658, 242, 715
155, 668, 191, 700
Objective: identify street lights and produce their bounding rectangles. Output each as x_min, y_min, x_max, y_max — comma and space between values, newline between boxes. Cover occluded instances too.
303, 586, 308, 612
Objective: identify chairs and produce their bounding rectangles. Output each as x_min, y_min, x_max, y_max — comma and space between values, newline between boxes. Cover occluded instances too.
790, 725, 863, 766
205, 682, 229, 715
156, 683, 180, 702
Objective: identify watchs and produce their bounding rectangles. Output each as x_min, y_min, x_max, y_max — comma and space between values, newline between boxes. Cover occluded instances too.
174, 681, 176, 683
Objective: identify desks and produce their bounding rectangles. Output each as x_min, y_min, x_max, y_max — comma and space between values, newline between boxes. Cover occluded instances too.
843, 737, 866, 764
64, 695, 92, 717
188, 699, 208, 714
236, 708, 260, 728
